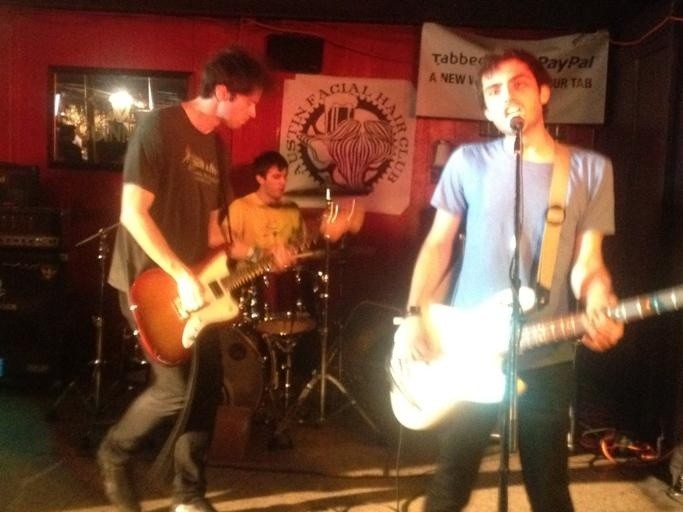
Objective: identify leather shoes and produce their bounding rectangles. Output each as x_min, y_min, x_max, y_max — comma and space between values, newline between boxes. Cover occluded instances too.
97, 444, 141, 512
170, 497, 218, 512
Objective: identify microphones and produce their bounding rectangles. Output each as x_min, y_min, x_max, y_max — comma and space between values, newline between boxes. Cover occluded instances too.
511, 116, 525, 155
324, 176, 332, 206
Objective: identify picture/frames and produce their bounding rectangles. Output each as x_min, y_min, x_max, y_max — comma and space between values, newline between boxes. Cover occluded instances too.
47, 64, 194, 174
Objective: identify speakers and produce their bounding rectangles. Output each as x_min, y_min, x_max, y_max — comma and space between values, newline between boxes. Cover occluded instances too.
263, 33, 323, 74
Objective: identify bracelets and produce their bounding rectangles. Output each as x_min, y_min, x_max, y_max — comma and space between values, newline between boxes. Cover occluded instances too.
247, 245, 262, 265
402, 304, 423, 318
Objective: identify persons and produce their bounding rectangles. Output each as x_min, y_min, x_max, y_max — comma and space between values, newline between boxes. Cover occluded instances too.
95, 43, 299, 512
392, 45, 624, 512
221, 147, 312, 282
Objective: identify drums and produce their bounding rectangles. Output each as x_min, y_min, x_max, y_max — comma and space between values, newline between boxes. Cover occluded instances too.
239, 265, 318, 336
217, 312, 294, 415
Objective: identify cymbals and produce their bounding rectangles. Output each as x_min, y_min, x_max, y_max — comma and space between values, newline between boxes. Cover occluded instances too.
282, 184, 373, 199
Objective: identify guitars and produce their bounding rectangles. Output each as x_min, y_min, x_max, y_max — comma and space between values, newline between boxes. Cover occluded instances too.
130, 204, 372, 366
391, 282, 683, 433
